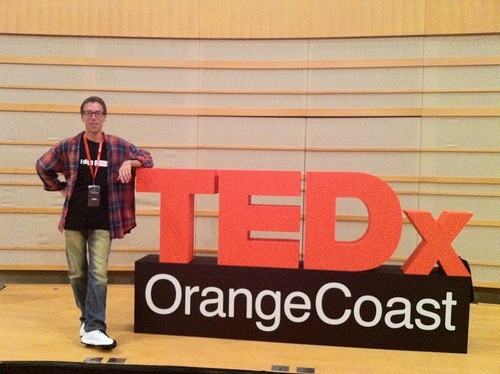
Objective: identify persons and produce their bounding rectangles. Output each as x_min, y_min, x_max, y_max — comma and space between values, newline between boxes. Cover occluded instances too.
35, 96, 154, 348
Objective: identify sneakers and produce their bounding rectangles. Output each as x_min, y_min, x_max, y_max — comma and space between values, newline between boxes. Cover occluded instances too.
80, 322, 117, 349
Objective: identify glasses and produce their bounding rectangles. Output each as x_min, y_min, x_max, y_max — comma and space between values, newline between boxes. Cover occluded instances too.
82, 109, 105, 118
